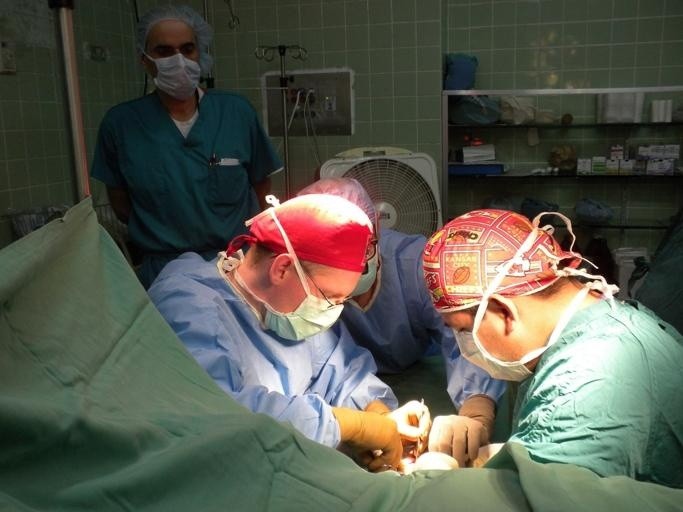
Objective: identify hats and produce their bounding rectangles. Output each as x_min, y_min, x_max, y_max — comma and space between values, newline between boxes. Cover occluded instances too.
136, 5, 213, 78
245, 178, 378, 273
421, 209, 583, 314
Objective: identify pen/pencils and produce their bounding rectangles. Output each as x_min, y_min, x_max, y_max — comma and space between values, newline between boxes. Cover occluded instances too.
212, 152, 216, 165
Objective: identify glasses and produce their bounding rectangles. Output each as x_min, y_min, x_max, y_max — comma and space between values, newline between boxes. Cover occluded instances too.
301, 264, 354, 308
364, 244, 376, 263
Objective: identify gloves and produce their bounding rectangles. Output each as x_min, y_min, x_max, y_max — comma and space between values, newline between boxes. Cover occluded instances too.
333, 397, 496, 472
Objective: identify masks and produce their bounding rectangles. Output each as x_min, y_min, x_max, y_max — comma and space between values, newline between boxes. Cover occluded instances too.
452, 299, 534, 382
255, 262, 345, 341
142, 50, 201, 96
349, 244, 378, 298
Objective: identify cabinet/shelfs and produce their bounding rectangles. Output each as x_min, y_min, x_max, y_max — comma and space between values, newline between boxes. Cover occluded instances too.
443, 86, 682, 230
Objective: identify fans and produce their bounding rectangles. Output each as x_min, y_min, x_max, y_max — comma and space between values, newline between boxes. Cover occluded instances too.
319, 150, 444, 240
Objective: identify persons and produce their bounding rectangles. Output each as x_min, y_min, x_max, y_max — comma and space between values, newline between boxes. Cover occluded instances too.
295, 177, 507, 468
90, 6, 282, 292
148, 194, 406, 474
422, 209, 683, 482
